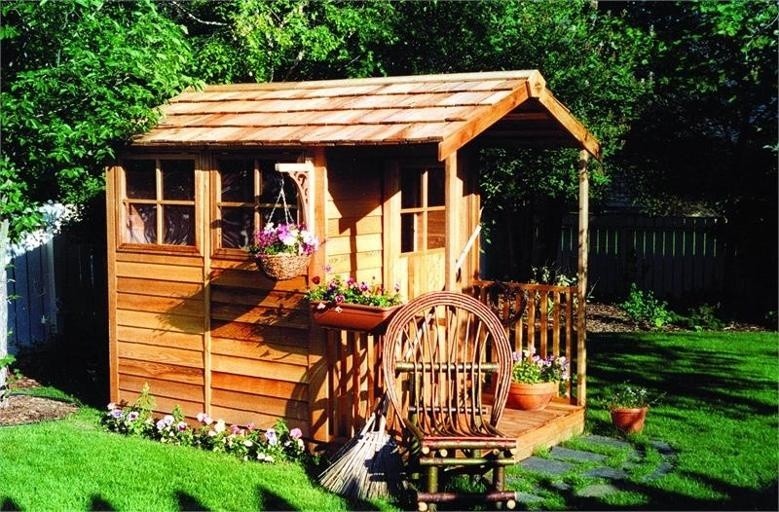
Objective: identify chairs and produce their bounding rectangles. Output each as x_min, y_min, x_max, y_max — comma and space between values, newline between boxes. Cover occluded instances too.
382, 290, 517, 512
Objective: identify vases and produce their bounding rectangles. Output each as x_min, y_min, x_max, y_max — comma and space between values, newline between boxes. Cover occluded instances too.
308, 301, 405, 333
611, 407, 648, 433
498, 382, 555, 411
255, 253, 311, 281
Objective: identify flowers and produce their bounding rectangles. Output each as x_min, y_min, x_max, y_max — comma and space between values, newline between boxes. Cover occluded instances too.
300, 263, 404, 313
511, 345, 569, 384
604, 382, 667, 409
249, 223, 320, 258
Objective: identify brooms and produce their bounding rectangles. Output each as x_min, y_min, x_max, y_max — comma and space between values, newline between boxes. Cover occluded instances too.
318, 224, 484, 500
